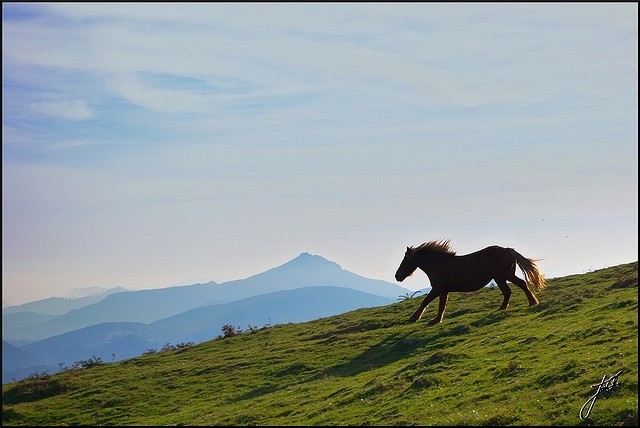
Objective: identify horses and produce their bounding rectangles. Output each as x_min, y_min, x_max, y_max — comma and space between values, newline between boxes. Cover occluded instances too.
395, 238, 547, 324
